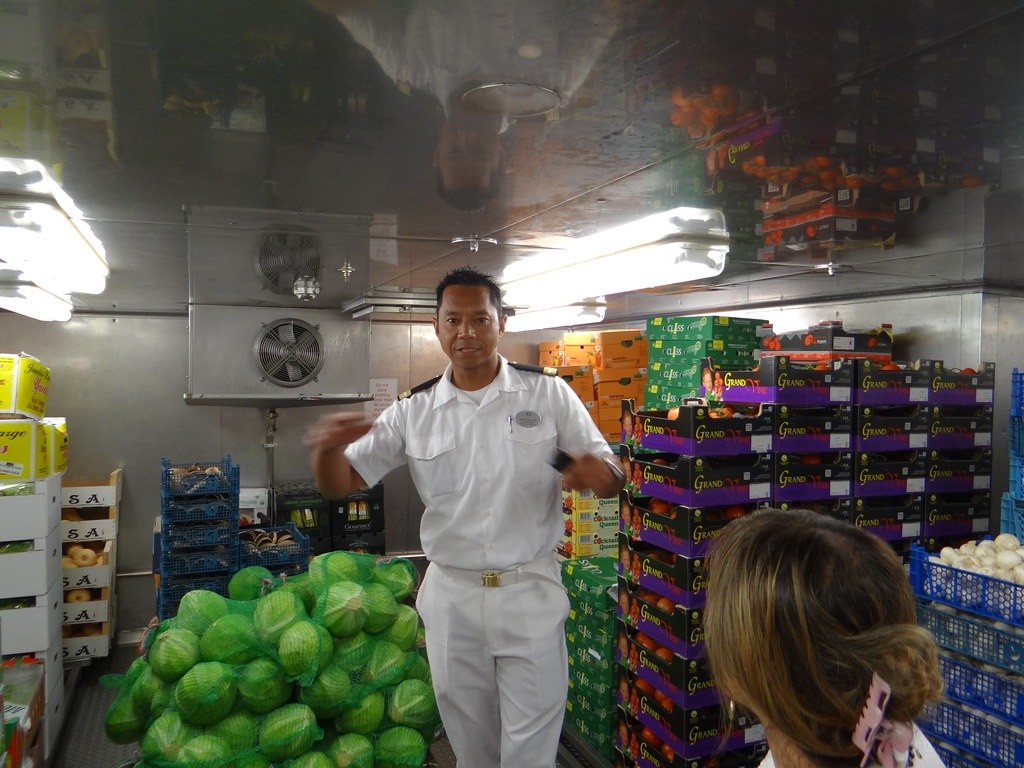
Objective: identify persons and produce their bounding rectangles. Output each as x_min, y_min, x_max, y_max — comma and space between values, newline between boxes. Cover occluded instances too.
617, 631, 637, 672
702, 507, 947, 768
619, 547, 639, 583
622, 456, 641, 493
618, 676, 638, 717
302, 268, 626, 768
623, 410, 641, 447
618, 587, 639, 628
702, 367, 724, 401
620, 501, 640, 537
619, 719, 638, 761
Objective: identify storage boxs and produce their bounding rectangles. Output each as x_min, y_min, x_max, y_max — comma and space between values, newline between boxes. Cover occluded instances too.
539, 316, 1024, 767
0, 352, 69, 484
152, 458, 387, 622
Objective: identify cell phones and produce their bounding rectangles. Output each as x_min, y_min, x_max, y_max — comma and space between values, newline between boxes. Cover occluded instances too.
548, 447, 574, 472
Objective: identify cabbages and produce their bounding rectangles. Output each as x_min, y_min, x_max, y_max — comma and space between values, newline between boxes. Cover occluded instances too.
104, 550, 440, 768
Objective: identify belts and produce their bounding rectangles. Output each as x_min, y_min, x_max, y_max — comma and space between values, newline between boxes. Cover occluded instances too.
438, 550, 568, 593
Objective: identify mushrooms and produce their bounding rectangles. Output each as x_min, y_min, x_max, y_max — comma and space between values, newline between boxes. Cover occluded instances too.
923, 534, 1024, 768
167, 463, 296, 593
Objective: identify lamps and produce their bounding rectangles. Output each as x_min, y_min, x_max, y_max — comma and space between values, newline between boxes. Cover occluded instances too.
497, 207, 730, 333
0, 157, 111, 323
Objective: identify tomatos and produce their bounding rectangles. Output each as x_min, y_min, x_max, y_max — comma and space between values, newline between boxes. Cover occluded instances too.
628, 406, 735, 761
668, 84, 980, 193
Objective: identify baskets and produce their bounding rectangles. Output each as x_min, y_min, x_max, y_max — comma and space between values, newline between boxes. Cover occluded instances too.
152, 454, 309, 622
909, 367, 1024, 768
